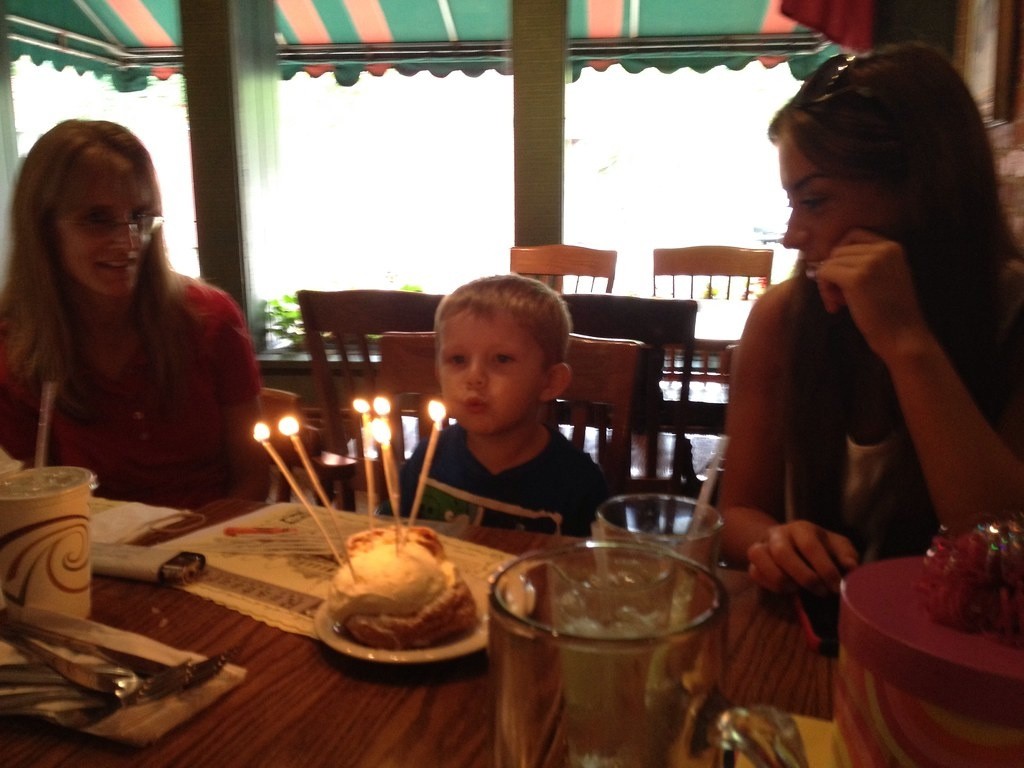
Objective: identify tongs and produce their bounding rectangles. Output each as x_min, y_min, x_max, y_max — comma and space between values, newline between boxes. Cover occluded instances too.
1, 620, 195, 697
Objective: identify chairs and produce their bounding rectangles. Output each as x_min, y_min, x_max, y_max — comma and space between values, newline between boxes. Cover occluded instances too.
258, 385, 306, 503
365, 326, 646, 535
293, 289, 444, 520
650, 245, 774, 300
553, 291, 699, 536
507, 243, 618, 294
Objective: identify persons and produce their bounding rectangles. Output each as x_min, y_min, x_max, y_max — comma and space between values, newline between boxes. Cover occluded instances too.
708, 43, 1022, 595
376, 272, 610, 539
0, 121, 276, 509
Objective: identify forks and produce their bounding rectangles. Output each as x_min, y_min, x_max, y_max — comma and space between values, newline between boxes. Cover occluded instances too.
0, 615, 231, 708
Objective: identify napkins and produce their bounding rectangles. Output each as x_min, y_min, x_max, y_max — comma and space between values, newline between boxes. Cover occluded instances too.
0, 605, 247, 743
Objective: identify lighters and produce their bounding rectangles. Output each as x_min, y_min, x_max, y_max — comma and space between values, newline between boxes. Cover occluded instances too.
90, 536, 207, 586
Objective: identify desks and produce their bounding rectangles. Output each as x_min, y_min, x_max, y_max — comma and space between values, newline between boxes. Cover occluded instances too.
662, 302, 753, 381
555, 382, 731, 496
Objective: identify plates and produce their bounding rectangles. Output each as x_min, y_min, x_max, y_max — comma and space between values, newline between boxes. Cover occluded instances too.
0, 619, 247, 743
315, 573, 492, 662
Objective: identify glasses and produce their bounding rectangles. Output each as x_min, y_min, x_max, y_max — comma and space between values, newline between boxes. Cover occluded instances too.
790, 52, 901, 135
55, 208, 165, 236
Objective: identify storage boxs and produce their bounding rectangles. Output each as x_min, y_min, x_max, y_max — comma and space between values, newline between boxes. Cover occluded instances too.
834, 557, 1024, 768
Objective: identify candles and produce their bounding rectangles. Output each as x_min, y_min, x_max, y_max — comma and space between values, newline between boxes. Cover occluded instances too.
253, 395, 447, 585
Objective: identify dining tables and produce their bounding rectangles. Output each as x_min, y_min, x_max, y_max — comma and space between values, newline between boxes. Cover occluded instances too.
0, 492, 834, 768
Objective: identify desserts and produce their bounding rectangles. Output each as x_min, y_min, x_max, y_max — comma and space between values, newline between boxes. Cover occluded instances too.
328, 523, 478, 647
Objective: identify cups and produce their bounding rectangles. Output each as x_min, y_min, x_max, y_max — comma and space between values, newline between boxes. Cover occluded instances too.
0, 466, 94, 620
596, 493, 722, 583
486, 540, 808, 768
